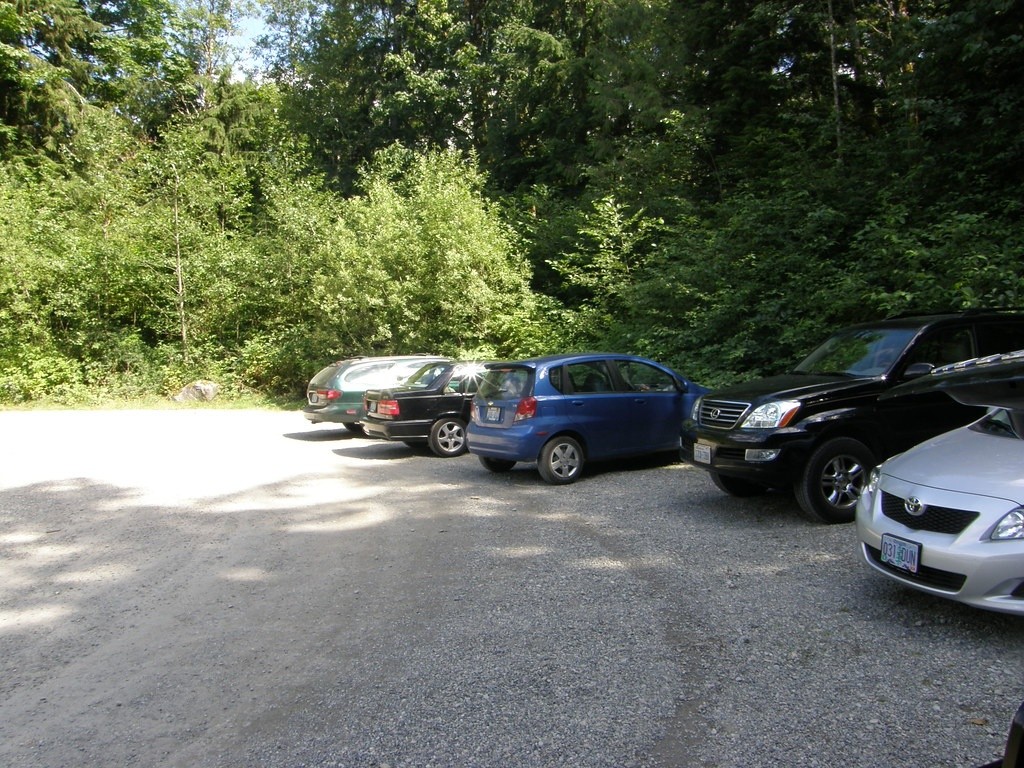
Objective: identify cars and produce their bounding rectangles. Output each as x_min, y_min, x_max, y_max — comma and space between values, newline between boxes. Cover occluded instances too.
359, 359, 519, 458
464, 354, 720, 485
853, 345, 1024, 617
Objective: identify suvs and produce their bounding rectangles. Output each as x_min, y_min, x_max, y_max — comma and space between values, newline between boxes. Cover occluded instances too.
679, 304, 1024, 525
301, 352, 452, 431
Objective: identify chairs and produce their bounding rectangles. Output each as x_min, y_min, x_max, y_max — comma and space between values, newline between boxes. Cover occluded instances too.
584, 373, 607, 392
936, 333, 969, 367
